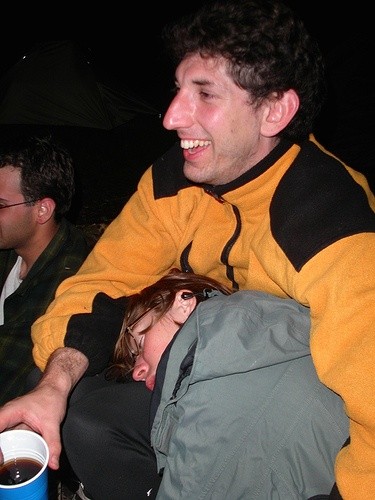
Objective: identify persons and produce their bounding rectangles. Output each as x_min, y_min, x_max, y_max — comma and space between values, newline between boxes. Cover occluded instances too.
0, 0, 374, 500
115, 269, 351, 500
0, 134, 108, 408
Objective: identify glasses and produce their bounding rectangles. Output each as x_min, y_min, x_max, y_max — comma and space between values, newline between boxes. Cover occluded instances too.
0, 198, 41, 209
123, 301, 162, 362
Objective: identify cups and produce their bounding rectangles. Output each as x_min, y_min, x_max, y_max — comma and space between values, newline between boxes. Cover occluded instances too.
0, 429, 48, 500
